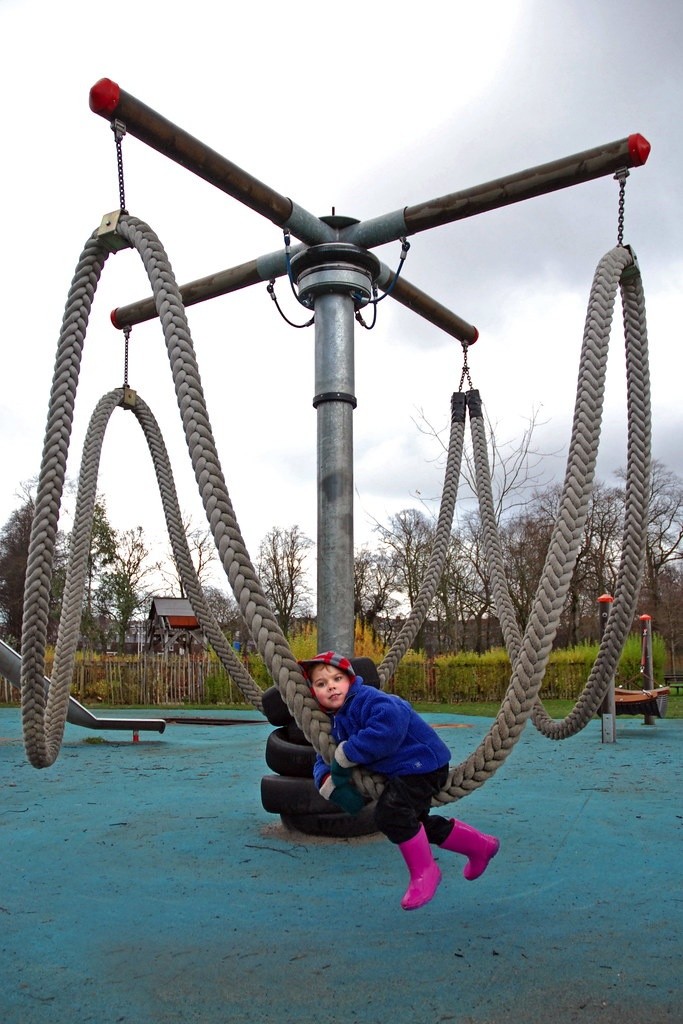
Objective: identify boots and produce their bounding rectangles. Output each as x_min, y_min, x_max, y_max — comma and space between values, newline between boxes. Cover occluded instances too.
398, 821, 442, 910
439, 817, 500, 880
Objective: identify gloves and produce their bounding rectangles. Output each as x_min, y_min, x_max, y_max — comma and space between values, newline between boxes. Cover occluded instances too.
318, 775, 366, 817
329, 741, 357, 788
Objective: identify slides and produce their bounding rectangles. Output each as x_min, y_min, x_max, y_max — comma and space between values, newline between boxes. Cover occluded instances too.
0, 640, 167, 735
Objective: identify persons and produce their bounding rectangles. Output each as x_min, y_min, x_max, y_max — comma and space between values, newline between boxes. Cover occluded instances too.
299, 652, 500, 910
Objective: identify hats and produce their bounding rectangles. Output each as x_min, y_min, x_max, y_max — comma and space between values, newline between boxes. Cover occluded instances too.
297, 651, 356, 712
